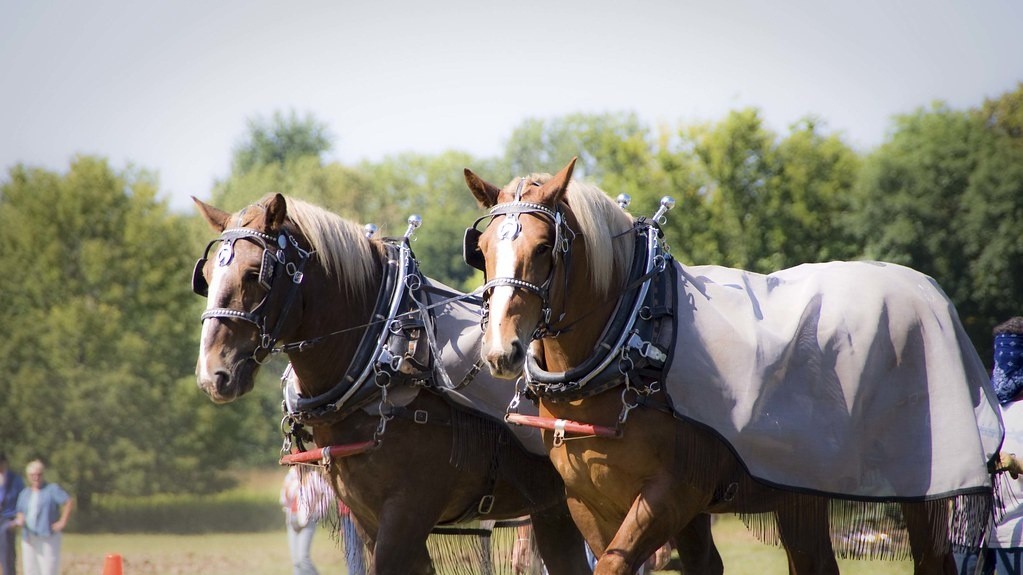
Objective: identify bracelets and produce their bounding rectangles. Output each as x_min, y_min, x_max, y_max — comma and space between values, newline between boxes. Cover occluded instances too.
516, 538, 529, 542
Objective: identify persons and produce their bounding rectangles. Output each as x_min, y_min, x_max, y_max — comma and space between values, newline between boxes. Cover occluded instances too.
947, 316, 1023, 575
279, 448, 367, 575
1, 451, 74, 575
511, 515, 671, 575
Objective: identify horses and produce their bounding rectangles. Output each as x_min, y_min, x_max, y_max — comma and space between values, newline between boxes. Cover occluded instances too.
191, 192, 725, 575
463, 155, 1023, 575
